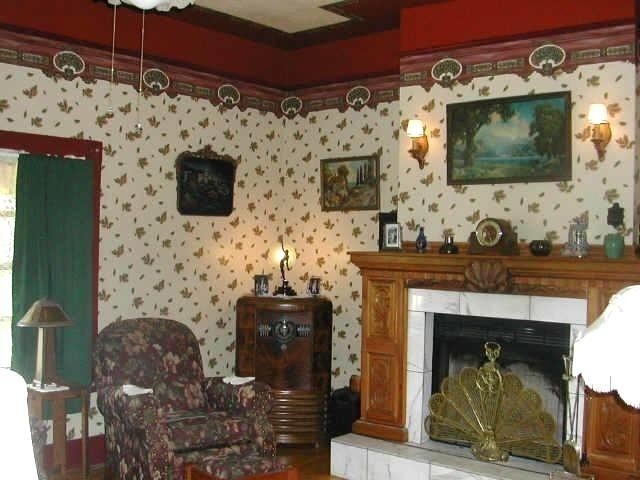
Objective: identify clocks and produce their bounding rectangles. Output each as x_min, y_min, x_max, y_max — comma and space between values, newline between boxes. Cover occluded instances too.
468, 217, 519, 254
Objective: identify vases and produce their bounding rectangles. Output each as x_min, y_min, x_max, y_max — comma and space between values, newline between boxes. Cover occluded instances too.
415, 225, 427, 249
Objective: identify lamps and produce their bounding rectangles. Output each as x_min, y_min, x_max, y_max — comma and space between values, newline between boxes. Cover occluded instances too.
583, 102, 616, 160
108, 0, 196, 129
406, 117, 429, 171
16, 299, 74, 388
571, 285, 640, 409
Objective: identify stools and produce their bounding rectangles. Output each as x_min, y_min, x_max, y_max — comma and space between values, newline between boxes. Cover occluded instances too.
186, 452, 297, 478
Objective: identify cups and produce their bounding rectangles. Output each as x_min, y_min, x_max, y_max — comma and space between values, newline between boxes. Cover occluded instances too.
530, 240, 551, 257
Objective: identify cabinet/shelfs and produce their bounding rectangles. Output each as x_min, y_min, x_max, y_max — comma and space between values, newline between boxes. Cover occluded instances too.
235, 297, 334, 447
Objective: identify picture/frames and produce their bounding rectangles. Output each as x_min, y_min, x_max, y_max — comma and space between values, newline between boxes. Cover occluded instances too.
306, 276, 321, 296
384, 223, 401, 248
253, 274, 270, 296
320, 153, 379, 214
442, 91, 574, 187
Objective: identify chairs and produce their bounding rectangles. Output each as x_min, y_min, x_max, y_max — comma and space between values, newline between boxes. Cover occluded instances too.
91, 315, 279, 478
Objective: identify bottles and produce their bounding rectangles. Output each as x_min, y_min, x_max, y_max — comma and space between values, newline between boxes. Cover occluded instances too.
605, 234, 624, 258
416, 227, 426, 249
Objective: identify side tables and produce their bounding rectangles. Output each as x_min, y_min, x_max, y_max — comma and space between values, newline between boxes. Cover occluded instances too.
27, 380, 89, 479
